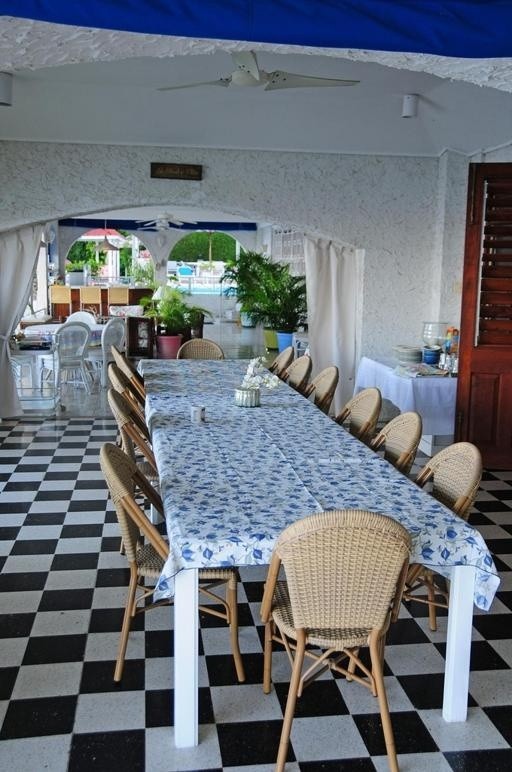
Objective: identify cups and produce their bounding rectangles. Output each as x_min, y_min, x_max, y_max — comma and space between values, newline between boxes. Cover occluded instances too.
189, 404, 206, 424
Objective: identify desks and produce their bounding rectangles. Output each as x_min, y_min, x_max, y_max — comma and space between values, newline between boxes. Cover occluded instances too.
355, 356, 458, 458
53, 284, 153, 320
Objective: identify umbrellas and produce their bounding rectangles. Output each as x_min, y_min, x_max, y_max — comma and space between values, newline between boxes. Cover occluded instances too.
76, 228, 125, 282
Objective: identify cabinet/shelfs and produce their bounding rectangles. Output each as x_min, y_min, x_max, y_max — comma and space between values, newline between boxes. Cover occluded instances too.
127, 316, 155, 358
10, 333, 66, 420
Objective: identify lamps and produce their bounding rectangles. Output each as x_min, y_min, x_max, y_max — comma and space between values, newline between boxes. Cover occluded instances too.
402, 94, 420, 119
93, 220, 120, 251
0, 70, 14, 107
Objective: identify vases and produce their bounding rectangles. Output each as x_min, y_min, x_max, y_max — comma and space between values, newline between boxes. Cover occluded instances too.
234, 386, 261, 407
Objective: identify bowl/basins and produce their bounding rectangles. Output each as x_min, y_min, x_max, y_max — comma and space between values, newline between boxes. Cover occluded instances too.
421, 345, 442, 365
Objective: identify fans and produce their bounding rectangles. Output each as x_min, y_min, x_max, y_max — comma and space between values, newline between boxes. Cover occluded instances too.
137, 223, 187, 232
135, 210, 197, 226
156, 49, 361, 92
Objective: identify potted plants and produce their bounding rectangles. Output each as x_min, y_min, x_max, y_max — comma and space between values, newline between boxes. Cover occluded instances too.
248, 250, 297, 349
188, 308, 204, 337
139, 286, 191, 359
218, 251, 273, 327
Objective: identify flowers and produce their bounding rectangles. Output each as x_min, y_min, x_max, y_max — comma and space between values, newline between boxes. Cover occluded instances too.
241, 356, 280, 390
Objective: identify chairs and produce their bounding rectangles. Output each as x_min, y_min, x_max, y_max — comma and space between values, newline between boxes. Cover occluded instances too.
110, 344, 147, 447
107, 388, 157, 555
107, 364, 146, 500
268, 345, 295, 376
336, 387, 382, 448
260, 510, 412, 772
106, 286, 129, 316
49, 286, 72, 316
86, 317, 126, 388
400, 442, 484, 632
66, 311, 95, 325
98, 442, 246, 683
370, 411, 422, 478
282, 355, 313, 394
303, 365, 340, 416
176, 338, 225, 359
35, 321, 93, 394
79, 286, 102, 316
